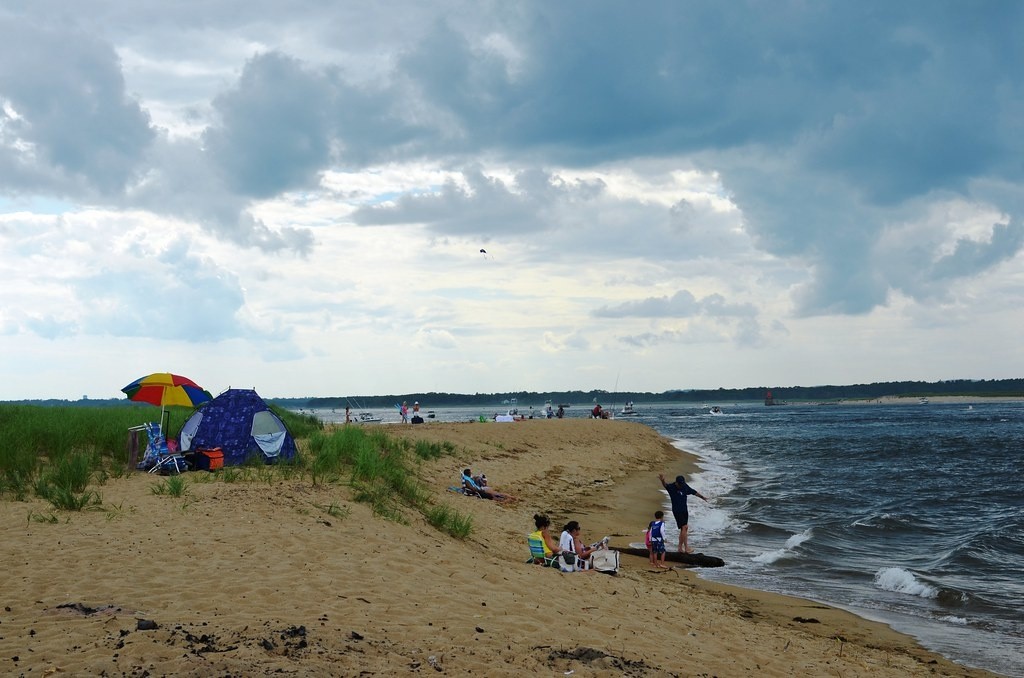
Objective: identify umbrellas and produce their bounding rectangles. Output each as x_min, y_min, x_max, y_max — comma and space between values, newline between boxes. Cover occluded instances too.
121, 373, 213, 437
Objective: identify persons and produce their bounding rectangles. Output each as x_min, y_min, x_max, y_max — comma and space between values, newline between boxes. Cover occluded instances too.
557, 407, 564, 418
412, 401, 420, 418
645, 511, 668, 569
564, 521, 597, 558
529, 513, 561, 558
626, 400, 634, 407
714, 407, 721, 413
493, 413, 498, 421
402, 402, 408, 424
463, 469, 517, 501
345, 407, 352, 425
657, 474, 709, 553
528, 406, 535, 419
594, 404, 608, 419
547, 406, 553, 419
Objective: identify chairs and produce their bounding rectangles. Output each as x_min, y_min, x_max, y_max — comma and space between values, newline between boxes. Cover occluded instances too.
527, 534, 559, 568
460, 470, 482, 499
128, 422, 195, 476
590, 409, 598, 418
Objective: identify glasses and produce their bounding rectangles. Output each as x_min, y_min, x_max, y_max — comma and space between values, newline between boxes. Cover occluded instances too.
578, 527, 580, 530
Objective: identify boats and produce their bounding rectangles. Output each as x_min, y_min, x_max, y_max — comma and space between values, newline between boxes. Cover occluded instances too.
426, 410, 436, 418
342, 412, 384, 425
918, 399, 929, 405
620, 405, 638, 416
710, 409, 725, 416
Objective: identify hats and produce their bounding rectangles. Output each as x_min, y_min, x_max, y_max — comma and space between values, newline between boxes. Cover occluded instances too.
676, 475, 685, 487
415, 402, 419, 404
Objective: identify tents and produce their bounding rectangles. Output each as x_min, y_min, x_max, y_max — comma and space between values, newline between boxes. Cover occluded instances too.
176, 387, 300, 466
